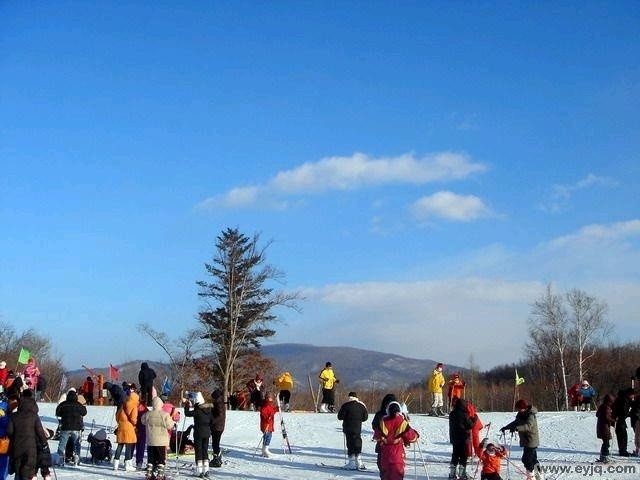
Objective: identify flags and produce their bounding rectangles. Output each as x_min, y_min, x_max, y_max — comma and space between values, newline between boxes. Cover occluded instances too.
514, 373, 526, 386
17, 345, 31, 365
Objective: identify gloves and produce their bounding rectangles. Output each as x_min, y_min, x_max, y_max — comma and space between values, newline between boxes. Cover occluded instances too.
501, 427, 517, 432
41, 442, 50, 454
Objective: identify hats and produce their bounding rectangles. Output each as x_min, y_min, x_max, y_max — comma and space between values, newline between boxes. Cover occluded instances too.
582, 380, 589, 386
94, 430, 106, 440
211, 389, 221, 399
484, 437, 498, 448
159, 393, 168, 402
66, 391, 78, 401
194, 392, 205, 406
436, 363, 444, 369
515, 399, 529, 409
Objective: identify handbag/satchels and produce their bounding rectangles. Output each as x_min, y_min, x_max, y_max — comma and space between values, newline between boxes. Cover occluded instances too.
0, 436, 10, 454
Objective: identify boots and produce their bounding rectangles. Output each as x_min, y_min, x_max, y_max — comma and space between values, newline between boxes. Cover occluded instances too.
262, 445, 272, 457
249, 403, 254, 411
574, 403, 591, 411
450, 464, 464, 478
526, 462, 544, 480
319, 403, 331, 412
429, 406, 445, 417
344, 453, 365, 470
58, 450, 224, 480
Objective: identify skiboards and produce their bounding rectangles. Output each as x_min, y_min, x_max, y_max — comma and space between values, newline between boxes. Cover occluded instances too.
587, 457, 640, 464
315, 463, 378, 473
51, 447, 240, 480
277, 392, 293, 453
416, 415, 449, 419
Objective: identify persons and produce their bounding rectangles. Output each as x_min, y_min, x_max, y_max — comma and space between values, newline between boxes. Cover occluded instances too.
318, 361, 340, 413
631, 367, 640, 458
613, 386, 634, 458
447, 398, 478, 480
372, 392, 399, 479
427, 361, 446, 417
594, 390, 616, 464
447, 374, 466, 412
474, 437, 507, 480
258, 390, 283, 458
246, 374, 266, 411
497, 399, 543, 480
568, 382, 584, 412
272, 368, 294, 413
0, 355, 248, 480
372, 400, 419, 480
578, 379, 596, 411
450, 396, 483, 463
336, 390, 369, 473
